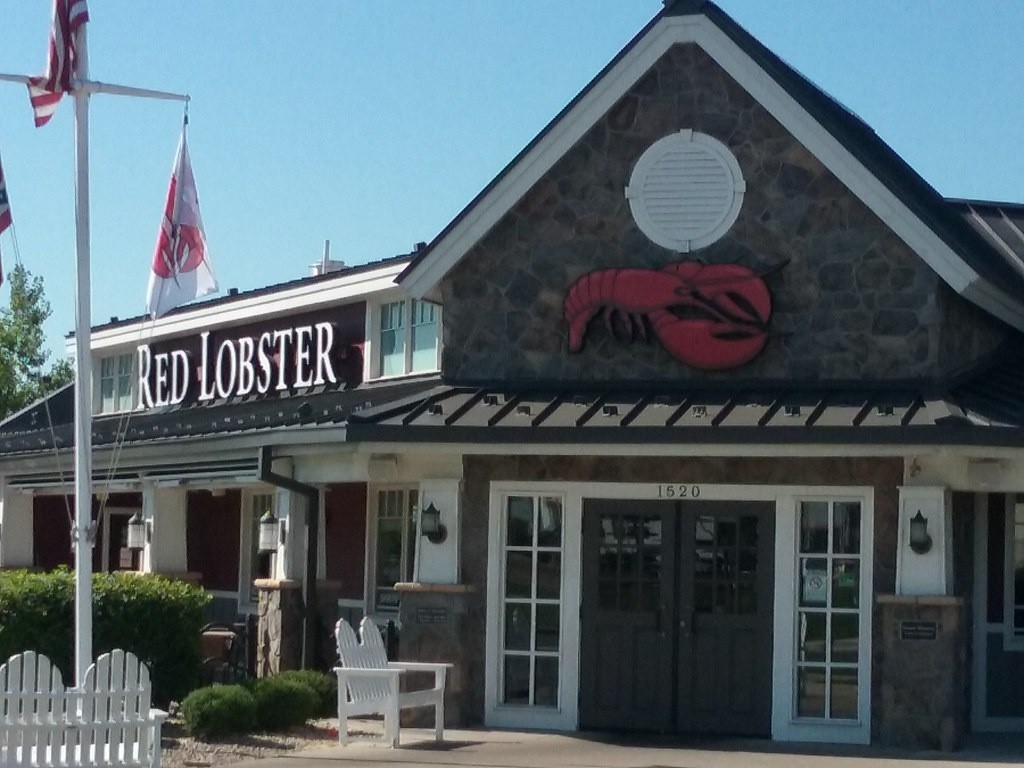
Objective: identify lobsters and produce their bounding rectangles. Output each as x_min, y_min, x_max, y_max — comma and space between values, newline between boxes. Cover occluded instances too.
564, 260, 769, 370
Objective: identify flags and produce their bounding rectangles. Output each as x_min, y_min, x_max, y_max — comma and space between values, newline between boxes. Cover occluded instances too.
26, 0, 90, 128
0, 158, 13, 285
144, 124, 218, 321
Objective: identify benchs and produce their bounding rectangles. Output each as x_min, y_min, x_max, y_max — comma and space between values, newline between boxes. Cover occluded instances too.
0, 648, 169, 768
333, 616, 455, 749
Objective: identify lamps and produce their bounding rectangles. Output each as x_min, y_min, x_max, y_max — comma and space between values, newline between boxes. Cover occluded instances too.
257, 508, 279, 555
909, 509, 932, 554
127, 511, 146, 551
421, 501, 447, 544
